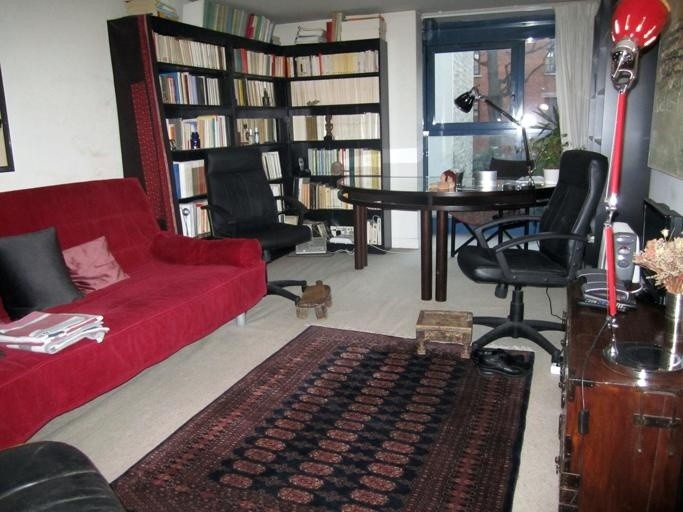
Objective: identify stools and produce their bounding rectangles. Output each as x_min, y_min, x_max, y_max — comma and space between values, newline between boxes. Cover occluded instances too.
295, 281, 331, 318
416, 310, 474, 360
0, 442, 127, 511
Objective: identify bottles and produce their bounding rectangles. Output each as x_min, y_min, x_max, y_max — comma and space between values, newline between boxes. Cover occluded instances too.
255, 127, 260, 144
191, 132, 200, 150
249, 129, 255, 145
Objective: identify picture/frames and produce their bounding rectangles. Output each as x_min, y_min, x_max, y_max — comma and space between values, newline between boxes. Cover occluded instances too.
0, 68, 15, 172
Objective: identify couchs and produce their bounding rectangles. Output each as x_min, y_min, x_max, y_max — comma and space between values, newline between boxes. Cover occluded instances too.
0, 178, 268, 448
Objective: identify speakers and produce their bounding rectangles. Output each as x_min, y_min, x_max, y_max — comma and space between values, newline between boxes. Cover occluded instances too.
596, 221, 640, 282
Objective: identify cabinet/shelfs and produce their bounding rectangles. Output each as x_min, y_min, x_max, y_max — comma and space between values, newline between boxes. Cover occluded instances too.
107, 14, 392, 254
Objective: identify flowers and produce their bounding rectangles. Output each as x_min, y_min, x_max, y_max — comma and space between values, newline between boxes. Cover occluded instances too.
630, 238, 682, 293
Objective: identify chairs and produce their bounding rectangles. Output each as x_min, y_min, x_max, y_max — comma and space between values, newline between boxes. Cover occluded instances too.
201, 147, 312, 305
450, 158, 534, 258
457, 151, 609, 365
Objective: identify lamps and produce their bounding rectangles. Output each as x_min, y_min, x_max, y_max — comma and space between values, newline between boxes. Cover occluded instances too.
454, 87, 531, 179
604, 0, 669, 329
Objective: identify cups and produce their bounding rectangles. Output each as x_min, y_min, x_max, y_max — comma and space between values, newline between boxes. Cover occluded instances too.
263, 96, 273, 105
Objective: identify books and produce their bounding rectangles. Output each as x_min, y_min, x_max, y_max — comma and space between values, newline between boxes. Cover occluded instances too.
291, 76, 380, 141
308, 147, 382, 175
0, 312, 108, 355
165, 114, 231, 151
234, 48, 379, 78
152, 30, 227, 71
159, 72, 221, 106
277, 213, 383, 246
235, 118, 278, 146
234, 78, 277, 107
261, 151, 286, 213
294, 176, 382, 210
171, 159, 211, 238
125, 2, 343, 45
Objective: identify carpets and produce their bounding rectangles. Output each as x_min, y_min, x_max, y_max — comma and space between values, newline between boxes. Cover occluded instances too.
110, 325, 535, 511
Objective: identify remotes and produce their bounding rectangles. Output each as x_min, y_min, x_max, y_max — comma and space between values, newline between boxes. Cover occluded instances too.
575, 297, 629, 313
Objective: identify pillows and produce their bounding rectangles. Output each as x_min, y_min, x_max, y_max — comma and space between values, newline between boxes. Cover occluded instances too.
0, 227, 83, 321
62, 236, 129, 296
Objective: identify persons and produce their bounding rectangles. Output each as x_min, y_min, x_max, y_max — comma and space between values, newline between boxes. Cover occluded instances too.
296, 155, 311, 177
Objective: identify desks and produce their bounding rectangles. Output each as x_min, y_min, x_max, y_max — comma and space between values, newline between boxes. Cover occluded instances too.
335, 177, 556, 301
556, 239, 683, 512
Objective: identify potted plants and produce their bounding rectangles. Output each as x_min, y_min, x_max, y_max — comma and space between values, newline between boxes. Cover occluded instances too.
532, 104, 563, 185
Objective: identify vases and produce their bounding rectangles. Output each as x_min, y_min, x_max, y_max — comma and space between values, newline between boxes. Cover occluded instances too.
666, 292, 683, 355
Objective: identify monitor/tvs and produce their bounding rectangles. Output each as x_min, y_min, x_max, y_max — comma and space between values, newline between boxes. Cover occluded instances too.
641, 197, 683, 304
